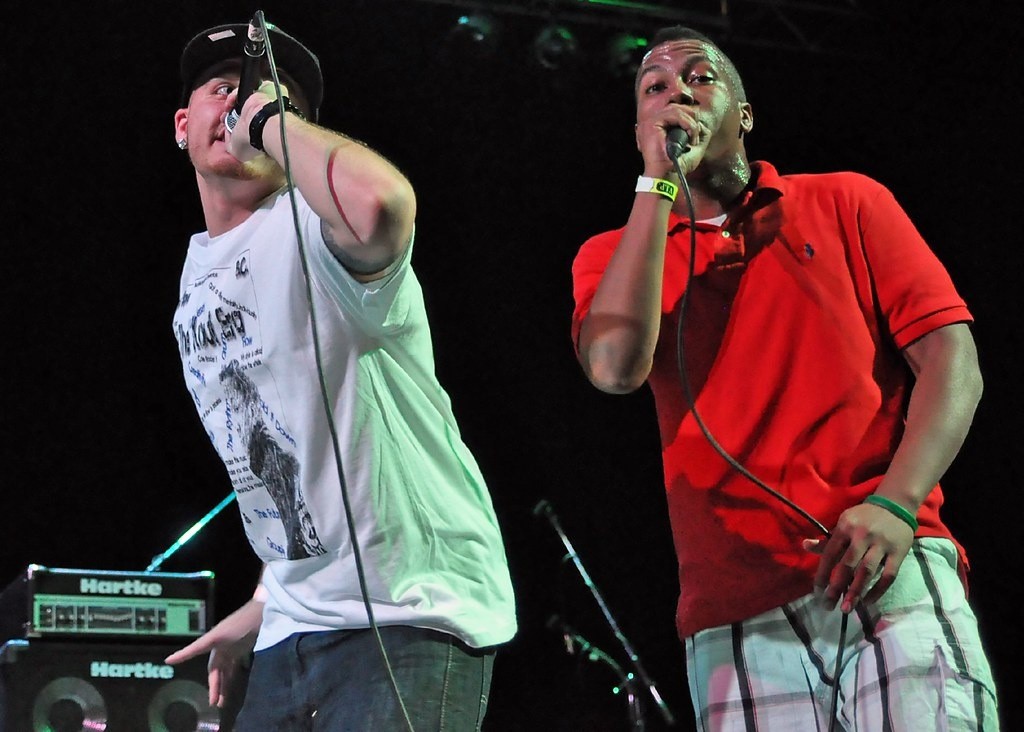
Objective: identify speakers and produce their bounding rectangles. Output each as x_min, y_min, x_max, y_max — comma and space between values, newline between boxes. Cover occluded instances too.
0, 640, 250, 732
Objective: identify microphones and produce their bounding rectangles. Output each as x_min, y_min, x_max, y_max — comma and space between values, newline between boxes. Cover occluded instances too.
665, 126, 689, 160
547, 615, 600, 662
225, 14, 266, 134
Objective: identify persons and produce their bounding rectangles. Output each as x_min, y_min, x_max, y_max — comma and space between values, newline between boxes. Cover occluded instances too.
164, 22, 519, 732
570, 24, 1001, 732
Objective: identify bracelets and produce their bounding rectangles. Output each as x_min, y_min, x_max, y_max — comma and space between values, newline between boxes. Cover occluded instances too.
635, 175, 679, 202
248, 96, 308, 153
864, 493, 919, 532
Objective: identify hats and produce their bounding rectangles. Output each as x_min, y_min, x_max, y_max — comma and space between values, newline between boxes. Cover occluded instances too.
179, 24, 323, 126
